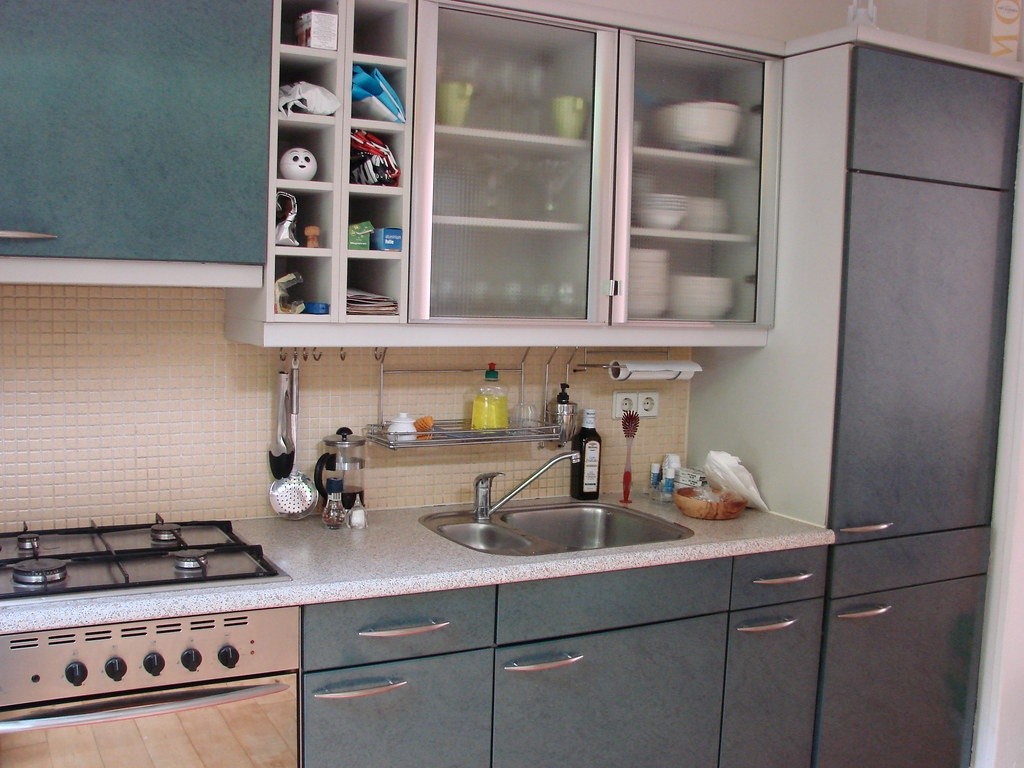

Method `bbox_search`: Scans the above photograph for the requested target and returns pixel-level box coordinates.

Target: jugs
[313,426,366,515]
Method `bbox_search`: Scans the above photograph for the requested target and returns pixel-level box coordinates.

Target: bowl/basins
[632,192,731,232]
[628,248,734,319]
[648,100,743,152]
[674,487,748,519]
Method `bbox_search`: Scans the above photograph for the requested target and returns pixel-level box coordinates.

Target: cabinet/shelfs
[0,0,1024,768]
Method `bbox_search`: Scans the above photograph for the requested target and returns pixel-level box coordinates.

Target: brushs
[622,410,640,502]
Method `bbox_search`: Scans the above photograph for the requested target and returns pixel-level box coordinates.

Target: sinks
[499,505,690,548]
[419,510,536,557]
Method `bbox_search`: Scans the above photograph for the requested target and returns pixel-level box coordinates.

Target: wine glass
[434,147,578,219]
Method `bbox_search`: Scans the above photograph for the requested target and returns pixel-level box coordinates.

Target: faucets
[470,448,582,520]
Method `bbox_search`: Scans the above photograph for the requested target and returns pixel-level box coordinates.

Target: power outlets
[637,389,659,419]
[612,391,638,419]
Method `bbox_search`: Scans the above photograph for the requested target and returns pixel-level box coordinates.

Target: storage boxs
[293,9,339,52]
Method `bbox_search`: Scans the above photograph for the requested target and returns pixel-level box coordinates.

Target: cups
[551,96,586,140]
[436,81,474,128]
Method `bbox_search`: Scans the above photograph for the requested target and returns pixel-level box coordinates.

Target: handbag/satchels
[278,82,341,117]
[351,64,405,125]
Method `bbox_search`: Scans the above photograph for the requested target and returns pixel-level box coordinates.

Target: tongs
[268,370,296,479]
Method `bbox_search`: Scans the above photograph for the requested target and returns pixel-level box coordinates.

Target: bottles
[347,494,369,529]
[387,411,418,443]
[321,476,346,530]
[650,462,661,487]
[569,408,602,500]
[664,469,675,493]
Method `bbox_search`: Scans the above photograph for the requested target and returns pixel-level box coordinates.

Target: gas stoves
[0,513,293,607]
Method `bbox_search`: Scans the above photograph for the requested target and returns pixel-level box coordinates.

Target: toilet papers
[607,359,704,381]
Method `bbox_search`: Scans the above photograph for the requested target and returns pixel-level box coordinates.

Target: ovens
[0,603,304,768]
